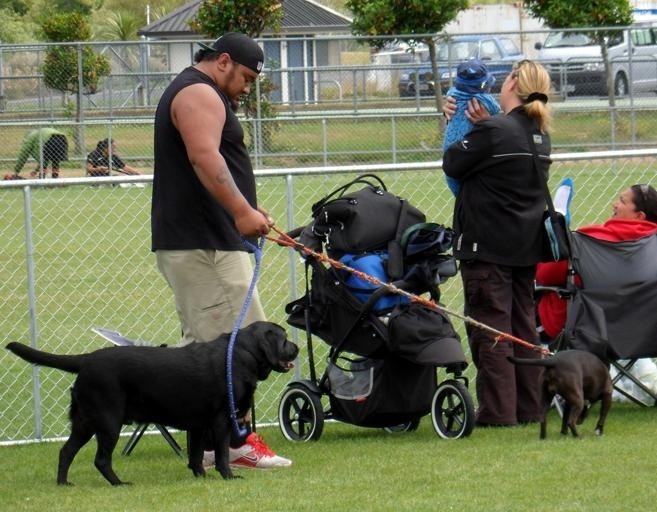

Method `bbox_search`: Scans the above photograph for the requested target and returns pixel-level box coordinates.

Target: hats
[452,58,497,93]
[195,30,264,75]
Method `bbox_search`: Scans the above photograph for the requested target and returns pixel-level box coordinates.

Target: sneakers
[201,432,292,471]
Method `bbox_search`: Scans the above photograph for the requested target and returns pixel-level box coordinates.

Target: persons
[535,181,657,340]
[12,126,70,178]
[86,138,142,186]
[151,31,294,469]
[445,58,503,197]
[440,60,556,428]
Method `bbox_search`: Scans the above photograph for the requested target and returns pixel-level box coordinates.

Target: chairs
[90,325,184,461]
[535,229,657,409]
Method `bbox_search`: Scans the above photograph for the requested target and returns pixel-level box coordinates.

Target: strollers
[278,225,477,446]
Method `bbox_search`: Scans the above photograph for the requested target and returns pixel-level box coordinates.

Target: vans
[533,20,657,96]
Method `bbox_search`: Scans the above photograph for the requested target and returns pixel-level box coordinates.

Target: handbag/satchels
[538,202,571,262]
[302,172,449,317]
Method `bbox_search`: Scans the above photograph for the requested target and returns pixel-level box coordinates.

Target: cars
[399,37,524,97]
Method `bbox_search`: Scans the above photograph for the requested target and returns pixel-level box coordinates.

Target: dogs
[4,320,299,487]
[505,349,614,440]
